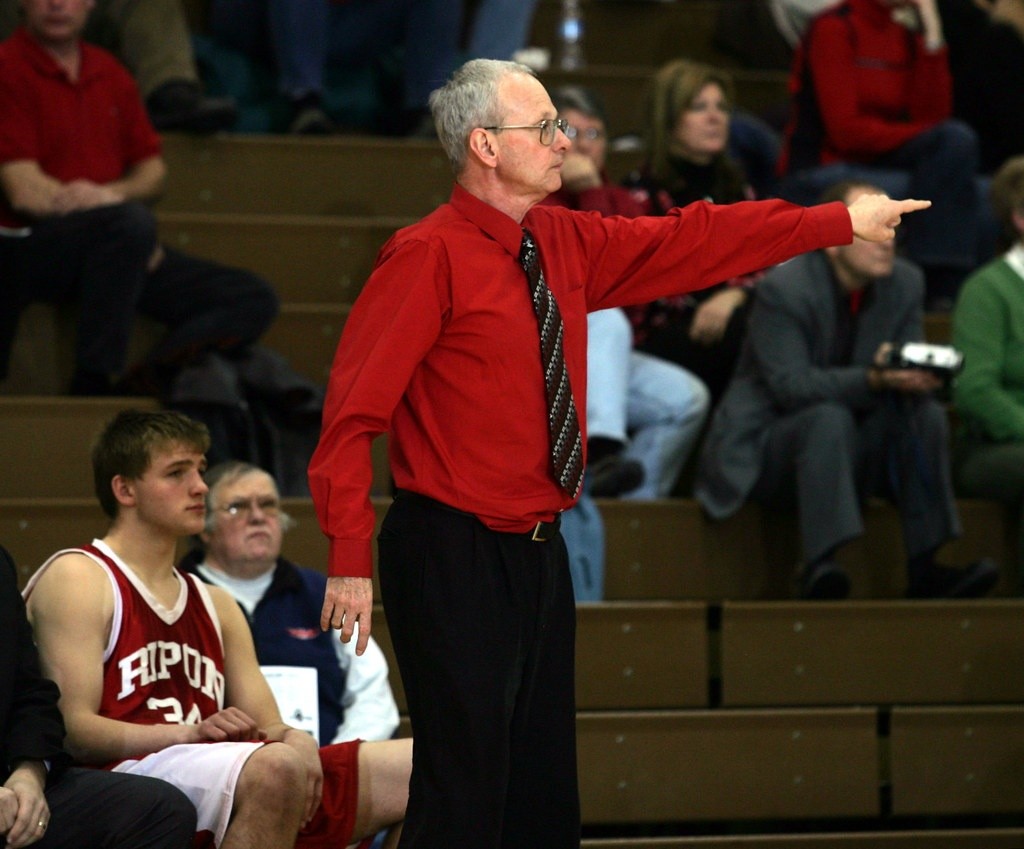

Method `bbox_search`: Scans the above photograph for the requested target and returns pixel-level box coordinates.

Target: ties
[520,225,584,500]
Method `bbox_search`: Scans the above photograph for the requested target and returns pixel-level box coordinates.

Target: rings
[38,821,46,829]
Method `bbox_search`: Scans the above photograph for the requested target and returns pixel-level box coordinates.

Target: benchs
[0,0,1024,849]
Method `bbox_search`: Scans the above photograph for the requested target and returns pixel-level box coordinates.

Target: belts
[530,518,562,542]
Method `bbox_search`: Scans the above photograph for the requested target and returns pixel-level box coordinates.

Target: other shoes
[903,558,1004,597]
[590,455,646,500]
[809,563,876,599]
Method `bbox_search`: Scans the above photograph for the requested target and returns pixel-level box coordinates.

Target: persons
[172,455,400,750]
[772,0,1024,320]
[535,79,711,501]
[0,0,278,390]
[107,1,227,134]
[307,57,935,849]
[249,0,537,134]
[941,158,1024,534]
[18,403,415,849]
[630,54,777,400]
[0,543,198,849]
[682,170,997,602]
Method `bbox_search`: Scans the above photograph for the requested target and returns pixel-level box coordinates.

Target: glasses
[479,118,570,146]
[209,500,281,521]
